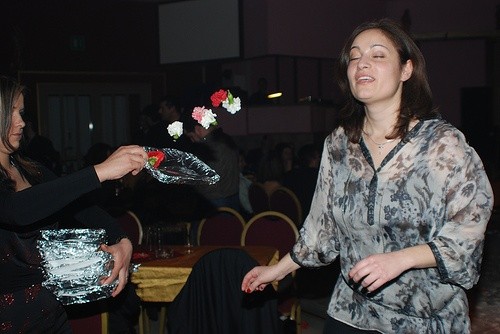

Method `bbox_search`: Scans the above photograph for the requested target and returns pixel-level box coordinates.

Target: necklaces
[366,133,399,155]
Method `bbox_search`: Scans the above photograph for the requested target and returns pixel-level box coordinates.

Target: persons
[27,92,318,198]
[242,20,495,334]
[0,72,148,334]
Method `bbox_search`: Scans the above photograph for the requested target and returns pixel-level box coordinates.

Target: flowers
[192,106,218,129]
[210,88,242,115]
[147,151,165,169]
[167,121,184,139]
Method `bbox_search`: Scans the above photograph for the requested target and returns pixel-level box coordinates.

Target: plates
[37,227,113,305]
[142,145,221,185]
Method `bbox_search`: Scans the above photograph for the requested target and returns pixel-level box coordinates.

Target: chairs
[67,298,108,334]
[118,183,302,334]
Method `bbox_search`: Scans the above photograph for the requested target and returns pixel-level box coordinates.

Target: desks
[129,243,280,334]
